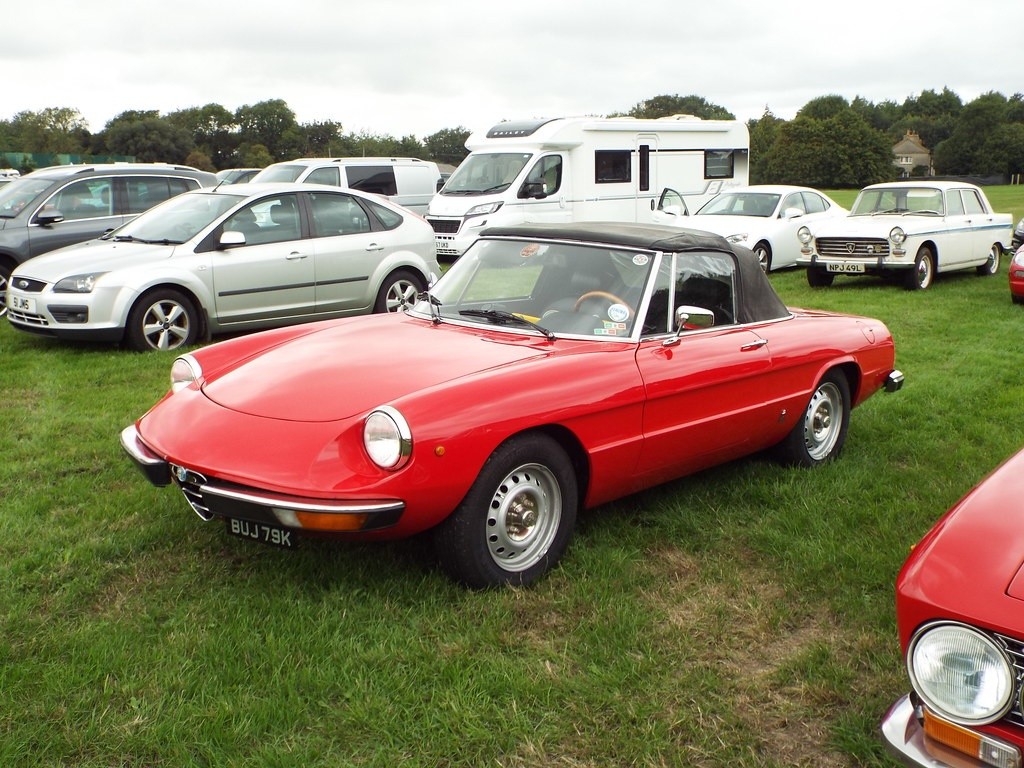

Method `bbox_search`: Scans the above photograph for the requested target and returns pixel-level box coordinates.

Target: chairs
[642,277,733,332]
[225,204,300,233]
[743,197,798,220]
[500,160,528,193]
[58,182,95,219]
[540,270,624,323]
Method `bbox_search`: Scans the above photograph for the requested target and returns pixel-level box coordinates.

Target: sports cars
[121,222,903,595]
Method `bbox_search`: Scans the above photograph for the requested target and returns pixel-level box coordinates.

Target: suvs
[0,162,221,308]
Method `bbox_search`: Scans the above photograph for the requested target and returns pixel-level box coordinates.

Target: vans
[213,167,292,187]
[229,157,445,228]
[418,113,750,255]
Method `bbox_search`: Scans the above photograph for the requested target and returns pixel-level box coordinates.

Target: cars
[75,184,148,212]
[0,168,20,178]
[791,181,1016,292]
[654,185,851,277]
[5,182,441,355]
[879,446,1024,768]
